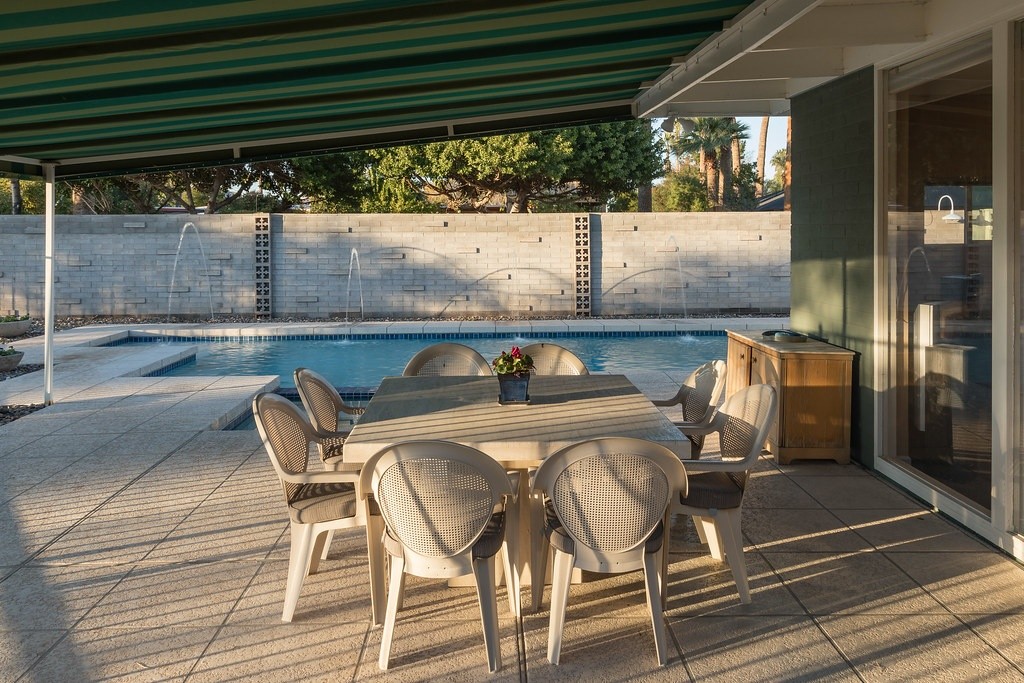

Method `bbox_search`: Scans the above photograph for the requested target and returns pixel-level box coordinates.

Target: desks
[343,375,691,586]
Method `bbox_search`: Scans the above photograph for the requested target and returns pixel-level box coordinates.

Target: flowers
[492,346,536,376]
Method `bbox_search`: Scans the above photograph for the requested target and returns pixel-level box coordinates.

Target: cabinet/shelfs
[725,329,856,465]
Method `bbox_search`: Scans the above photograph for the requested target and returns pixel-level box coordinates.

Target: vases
[496,370,531,407]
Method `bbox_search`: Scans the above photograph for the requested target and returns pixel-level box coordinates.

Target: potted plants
[0,315,32,338]
[0,346,24,371]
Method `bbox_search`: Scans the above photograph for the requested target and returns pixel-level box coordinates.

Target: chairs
[528,436,689,667]
[521,344,590,374]
[253,392,391,628]
[652,360,727,461]
[402,343,494,376]
[295,367,366,560]
[359,438,521,674]
[657,383,776,605]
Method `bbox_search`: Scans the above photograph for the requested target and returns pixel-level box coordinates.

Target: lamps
[938,195,962,224]
